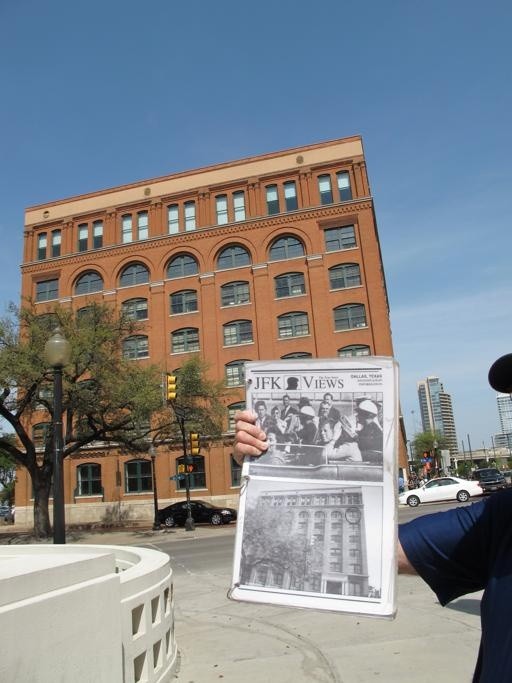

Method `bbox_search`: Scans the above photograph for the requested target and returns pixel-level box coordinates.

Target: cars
[471,468,512,492]
[398,477,483,507]
[160,500,237,526]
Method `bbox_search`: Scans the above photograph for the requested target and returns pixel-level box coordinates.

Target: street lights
[432,439,441,477]
[44,326,71,545]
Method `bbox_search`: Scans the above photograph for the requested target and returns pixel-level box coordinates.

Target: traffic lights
[164,373,179,402]
[190,433,202,457]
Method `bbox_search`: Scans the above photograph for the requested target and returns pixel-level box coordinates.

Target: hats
[489,353,512,393]
[354,400,378,415]
[299,406,316,416]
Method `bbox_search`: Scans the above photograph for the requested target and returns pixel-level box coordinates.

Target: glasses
[272,411,279,415]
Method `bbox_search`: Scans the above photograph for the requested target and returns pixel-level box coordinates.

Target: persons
[229,353,512,683]
[399,468,472,494]
[248,391,382,468]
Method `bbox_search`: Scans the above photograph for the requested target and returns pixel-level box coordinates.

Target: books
[228,357,401,622]
